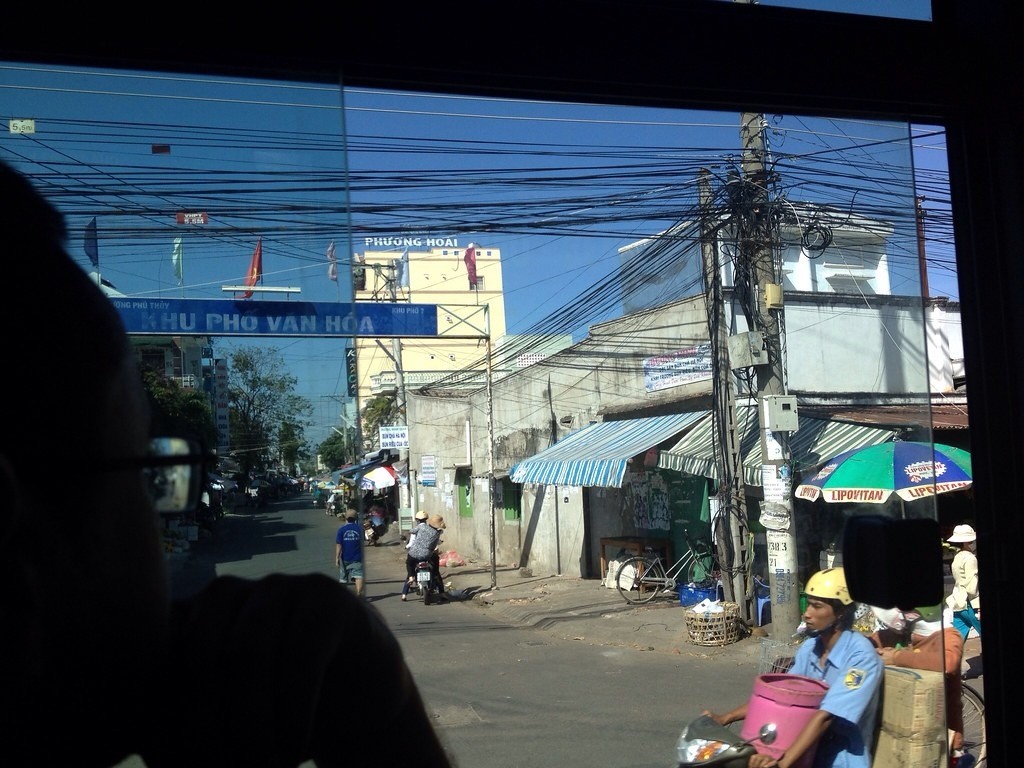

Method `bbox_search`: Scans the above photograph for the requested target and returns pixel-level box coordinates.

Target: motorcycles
[401,536,440,605]
[361,515,380,546]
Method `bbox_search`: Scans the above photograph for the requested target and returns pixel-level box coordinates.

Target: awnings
[658,406,895,488]
[509,410,712,488]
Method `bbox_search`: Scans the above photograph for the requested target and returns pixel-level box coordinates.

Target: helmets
[803,565,855,605]
[415,511,428,520]
[914,601,942,623]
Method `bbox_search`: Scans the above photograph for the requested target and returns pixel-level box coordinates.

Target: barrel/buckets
[741,673,829,767]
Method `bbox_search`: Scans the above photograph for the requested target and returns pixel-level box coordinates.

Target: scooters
[672,714,777,768]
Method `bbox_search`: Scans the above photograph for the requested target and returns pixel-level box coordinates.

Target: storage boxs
[871,727,949,768]
[677,582,724,608]
[881,664,947,742]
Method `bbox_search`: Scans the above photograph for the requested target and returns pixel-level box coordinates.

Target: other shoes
[407,580,413,585]
[401,596,406,601]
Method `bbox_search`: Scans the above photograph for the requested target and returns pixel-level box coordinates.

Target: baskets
[683,600,741,646]
[757,636,799,675]
[692,534,712,559]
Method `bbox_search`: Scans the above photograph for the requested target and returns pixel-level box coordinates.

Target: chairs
[755,576,771,627]
[398,508,412,535]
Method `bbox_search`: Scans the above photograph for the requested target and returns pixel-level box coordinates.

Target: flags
[236,238,262,298]
[172,237,183,278]
[397,250,409,287]
[84,217,98,266]
[464,248,476,284]
[327,242,337,281]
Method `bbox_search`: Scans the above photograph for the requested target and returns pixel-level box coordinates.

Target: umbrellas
[361,465,398,490]
[795,437,973,519]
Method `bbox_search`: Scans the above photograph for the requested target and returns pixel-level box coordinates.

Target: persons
[0,160,450,768]
[401,511,446,600]
[875,617,964,768]
[700,567,885,768]
[947,524,981,642]
[335,508,365,596]
[326,493,338,515]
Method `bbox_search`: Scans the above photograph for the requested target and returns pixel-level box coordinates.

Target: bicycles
[615,528,719,604]
[756,638,987,768]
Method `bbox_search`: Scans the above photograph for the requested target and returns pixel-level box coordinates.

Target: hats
[429,515,446,531]
[947,524,977,543]
[346,507,357,520]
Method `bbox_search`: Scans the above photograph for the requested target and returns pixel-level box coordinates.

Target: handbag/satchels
[945,585,968,612]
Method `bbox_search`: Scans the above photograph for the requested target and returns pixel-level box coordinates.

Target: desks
[599,537,673,595]
